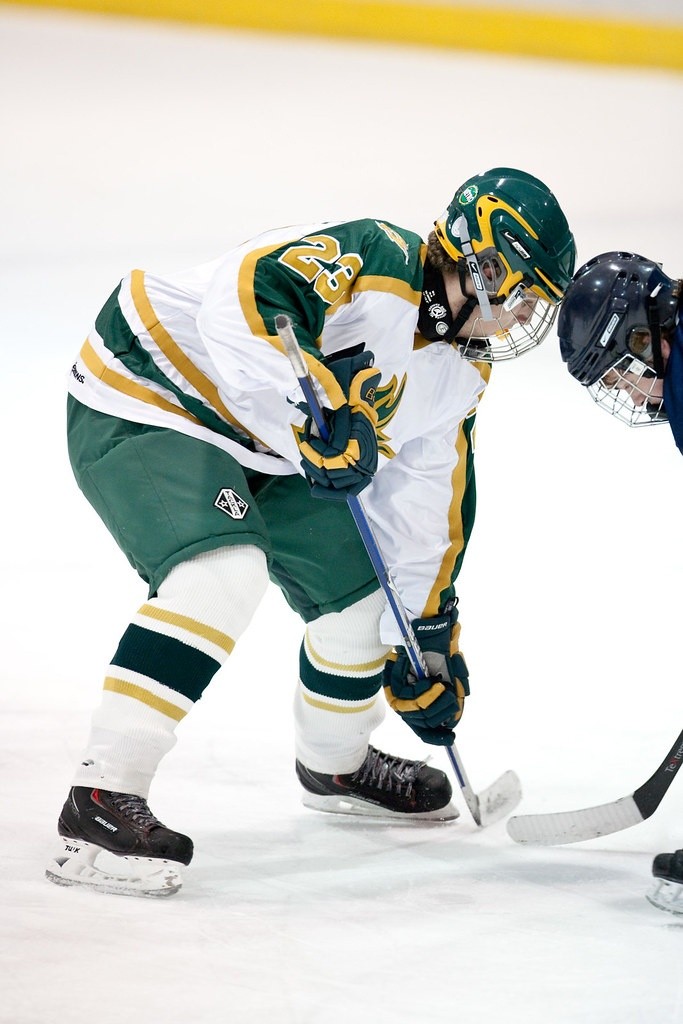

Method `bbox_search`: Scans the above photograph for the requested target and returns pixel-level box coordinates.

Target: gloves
[290,351,380,501]
[382,596,469,748]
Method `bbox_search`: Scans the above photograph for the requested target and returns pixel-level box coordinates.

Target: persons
[557,251,683,884]
[58,167,575,863]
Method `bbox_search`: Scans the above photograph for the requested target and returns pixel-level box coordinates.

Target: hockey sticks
[271,308,524,834]
[503,727,683,851]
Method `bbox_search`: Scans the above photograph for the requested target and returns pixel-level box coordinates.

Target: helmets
[436,168,576,362]
[557,251,680,428]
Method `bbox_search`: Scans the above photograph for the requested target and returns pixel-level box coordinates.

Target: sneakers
[45,787,193,900]
[296,745,460,821]
[646,850,683,918]
